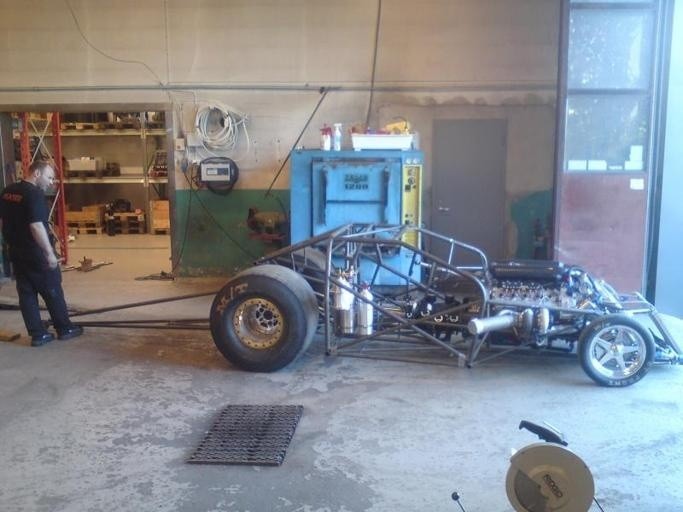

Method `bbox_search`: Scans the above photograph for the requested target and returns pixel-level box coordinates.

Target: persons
[0,160,83,347]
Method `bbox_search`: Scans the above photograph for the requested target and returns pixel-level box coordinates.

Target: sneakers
[31,330,54,346]
[58,325,83,340]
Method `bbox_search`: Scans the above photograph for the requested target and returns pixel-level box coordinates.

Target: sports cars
[208,219,683,388]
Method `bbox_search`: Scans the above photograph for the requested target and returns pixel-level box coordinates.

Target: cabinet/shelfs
[11,112,170,235]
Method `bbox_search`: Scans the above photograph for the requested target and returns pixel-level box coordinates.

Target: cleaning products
[334,123,341,151]
[320,124,332,151]
